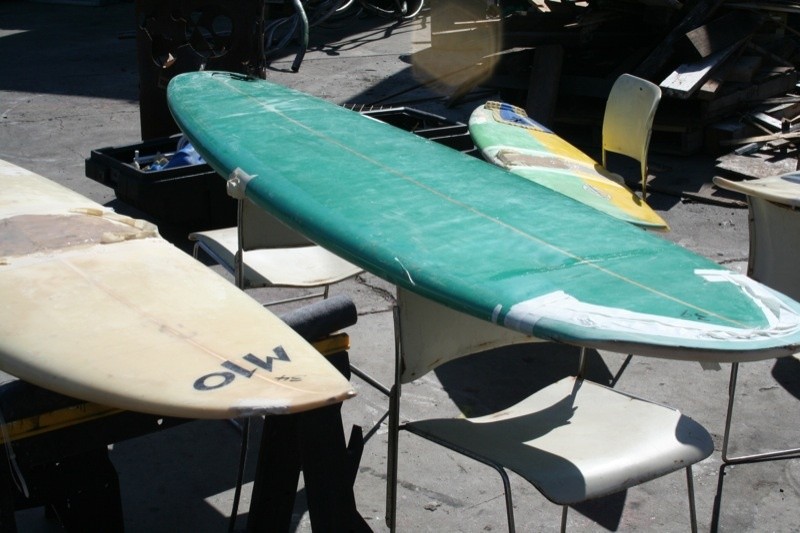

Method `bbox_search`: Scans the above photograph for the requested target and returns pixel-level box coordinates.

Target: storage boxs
[85,106,482,249]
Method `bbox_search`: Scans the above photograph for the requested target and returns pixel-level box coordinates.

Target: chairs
[384,283,714,533]
[603,73,662,201]
[189,174,366,304]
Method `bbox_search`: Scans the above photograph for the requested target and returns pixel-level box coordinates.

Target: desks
[713,169,800,303]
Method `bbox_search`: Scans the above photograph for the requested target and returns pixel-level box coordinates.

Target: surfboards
[167,71,800,363]
[1,156,359,419]
[468,102,668,232]
